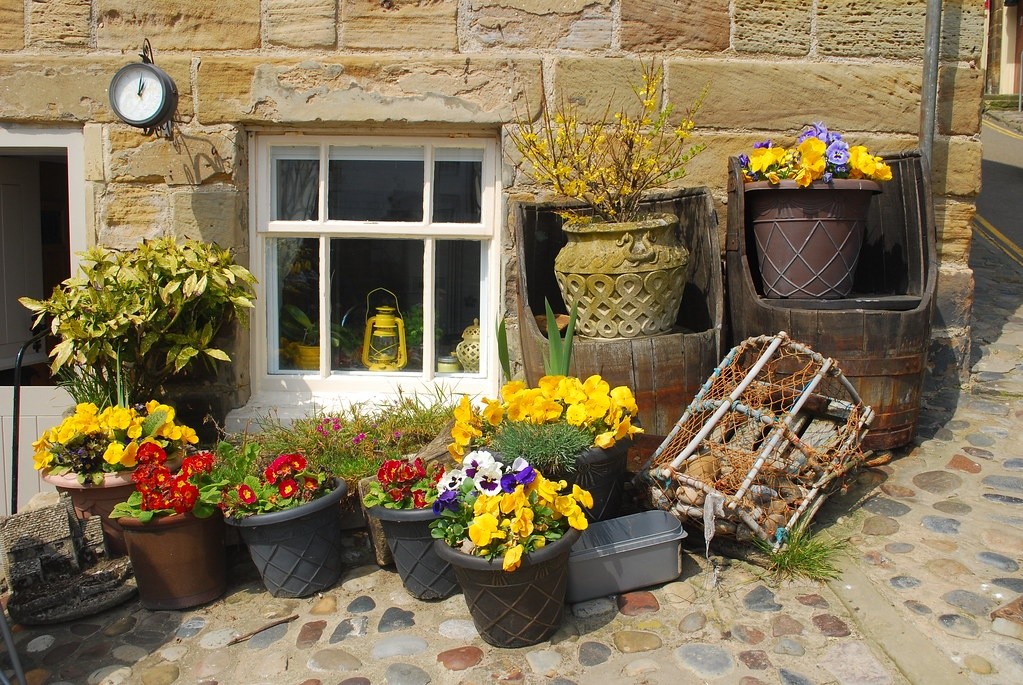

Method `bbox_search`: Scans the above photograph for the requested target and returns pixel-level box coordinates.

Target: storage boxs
[561,510,689,603]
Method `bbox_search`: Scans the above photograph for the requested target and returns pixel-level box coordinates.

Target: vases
[555,422,640,520]
[371,506,461,601]
[555,211,693,337]
[43,446,186,552]
[745,178,881,300]
[224,477,344,599]
[115,508,224,610]
[434,518,582,647]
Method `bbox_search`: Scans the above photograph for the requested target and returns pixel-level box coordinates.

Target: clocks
[107,63,179,131]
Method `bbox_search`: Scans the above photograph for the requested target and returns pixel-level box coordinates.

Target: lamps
[361,287,408,372]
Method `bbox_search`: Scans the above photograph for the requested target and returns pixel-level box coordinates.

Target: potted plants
[297,322,321,370]
[16,233,258,446]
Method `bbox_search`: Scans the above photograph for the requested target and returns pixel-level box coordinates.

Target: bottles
[437,356,459,373]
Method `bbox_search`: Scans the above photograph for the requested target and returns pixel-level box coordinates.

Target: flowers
[508,47,715,226]
[221,453,338,520]
[733,124,896,185]
[31,401,200,485]
[445,373,648,475]
[428,445,592,570]
[107,438,220,526]
[362,457,450,511]
